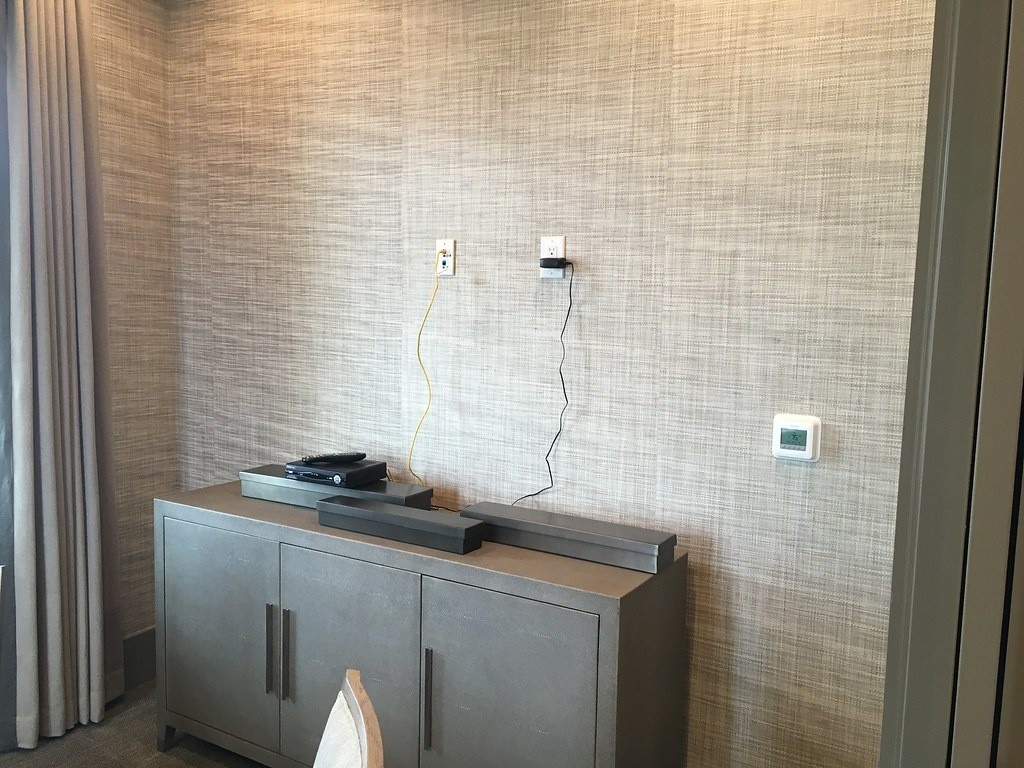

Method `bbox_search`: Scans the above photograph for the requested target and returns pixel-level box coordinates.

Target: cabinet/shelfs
[154,479,688,768]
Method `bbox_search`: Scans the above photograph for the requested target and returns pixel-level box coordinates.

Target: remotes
[302,452,367,464]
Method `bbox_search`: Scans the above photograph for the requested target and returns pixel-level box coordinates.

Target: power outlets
[540,235,566,279]
[435,238,455,276]
